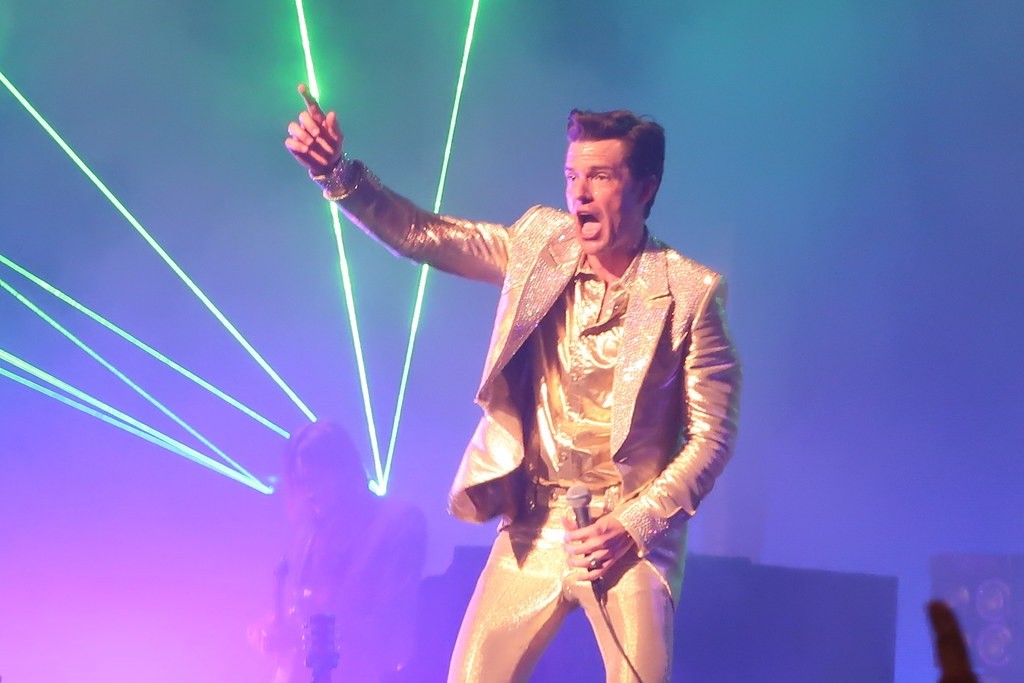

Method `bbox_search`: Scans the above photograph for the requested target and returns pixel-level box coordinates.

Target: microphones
[566,484,607,602]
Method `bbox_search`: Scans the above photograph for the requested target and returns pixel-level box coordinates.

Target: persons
[246,419,426,682]
[282,82,739,682]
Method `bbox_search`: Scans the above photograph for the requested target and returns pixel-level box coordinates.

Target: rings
[587,554,600,569]
